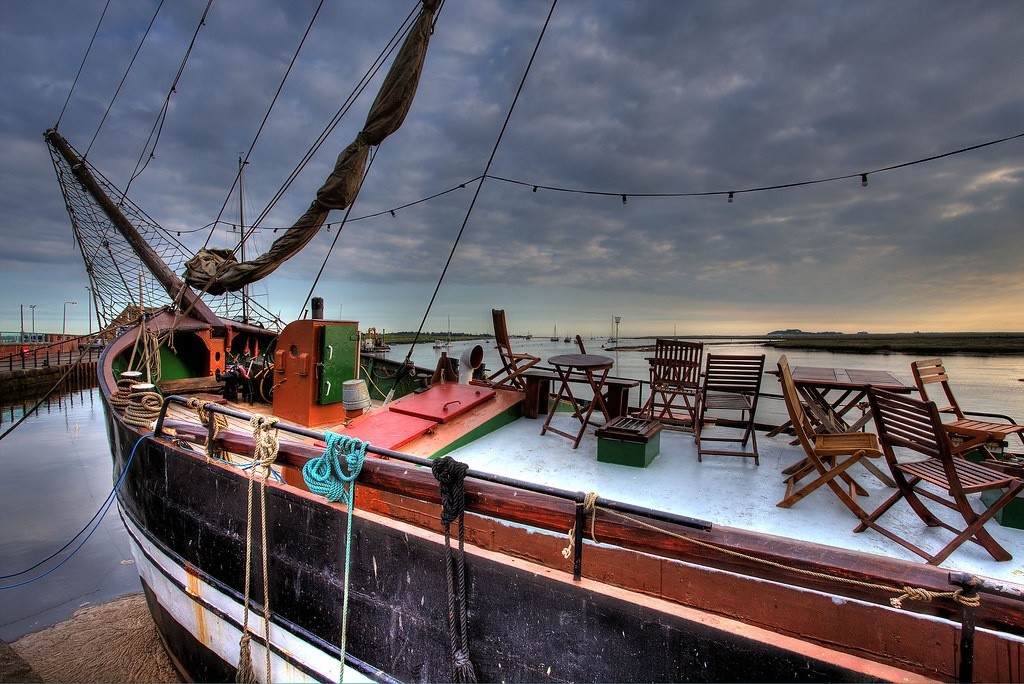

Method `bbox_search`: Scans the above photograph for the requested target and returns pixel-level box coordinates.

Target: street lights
[85,286,92,334]
[30,304,37,333]
[62,302,78,354]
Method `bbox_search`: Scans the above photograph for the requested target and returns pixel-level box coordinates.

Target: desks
[778,366,922,488]
[539,354,615,449]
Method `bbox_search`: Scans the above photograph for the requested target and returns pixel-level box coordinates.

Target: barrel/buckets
[341,379,373,420]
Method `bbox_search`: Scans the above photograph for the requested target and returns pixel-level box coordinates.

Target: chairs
[636,337,766,467]
[776,354,885,520]
[911,358,1024,498]
[853,384,1024,566]
[488,308,542,389]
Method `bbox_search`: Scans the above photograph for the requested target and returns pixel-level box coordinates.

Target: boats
[551,324,559,342]
[361,327,391,353]
[564,330,572,343]
[432,339,453,350]
[574,339,585,344]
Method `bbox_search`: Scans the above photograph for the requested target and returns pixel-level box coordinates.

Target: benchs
[522,369,640,422]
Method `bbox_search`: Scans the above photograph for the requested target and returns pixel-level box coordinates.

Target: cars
[78,333,109,352]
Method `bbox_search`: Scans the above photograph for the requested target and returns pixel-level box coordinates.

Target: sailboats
[42,2,1024,684]
[606,314,617,343]
